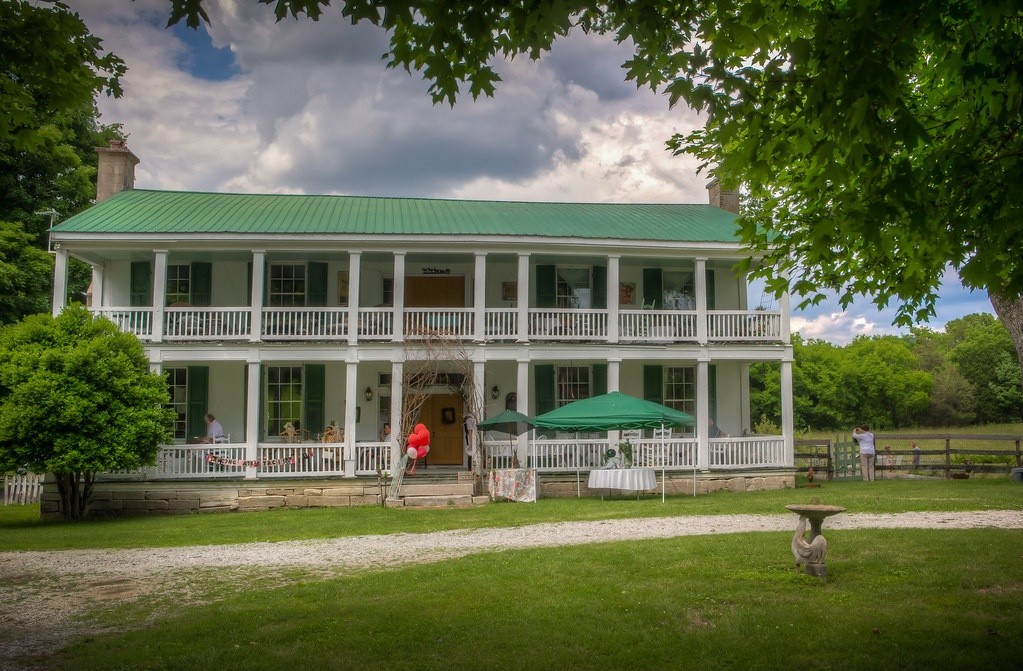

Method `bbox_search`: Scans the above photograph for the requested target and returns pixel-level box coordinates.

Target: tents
[534,392,697,504]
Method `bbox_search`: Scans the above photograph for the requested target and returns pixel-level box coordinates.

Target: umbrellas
[476,409,535,468]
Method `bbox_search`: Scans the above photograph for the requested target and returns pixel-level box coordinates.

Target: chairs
[483,435,495,441]
[212,434,231,444]
[279,427,342,443]
[622,429,641,439]
[653,428,672,439]
[537,434,547,440]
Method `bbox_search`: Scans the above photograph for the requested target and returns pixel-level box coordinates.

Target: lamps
[365,388,372,401]
[492,385,499,398]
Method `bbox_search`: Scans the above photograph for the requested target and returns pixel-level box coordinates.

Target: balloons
[407,423,430,460]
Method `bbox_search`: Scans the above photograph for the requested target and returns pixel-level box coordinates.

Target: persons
[202,412,225,444]
[911,440,920,471]
[358,424,391,470]
[852,425,875,483]
[883,445,895,473]
[676,418,727,457]
[320,426,333,442]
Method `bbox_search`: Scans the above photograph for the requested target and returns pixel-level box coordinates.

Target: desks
[588,468,657,501]
[487,468,539,503]
